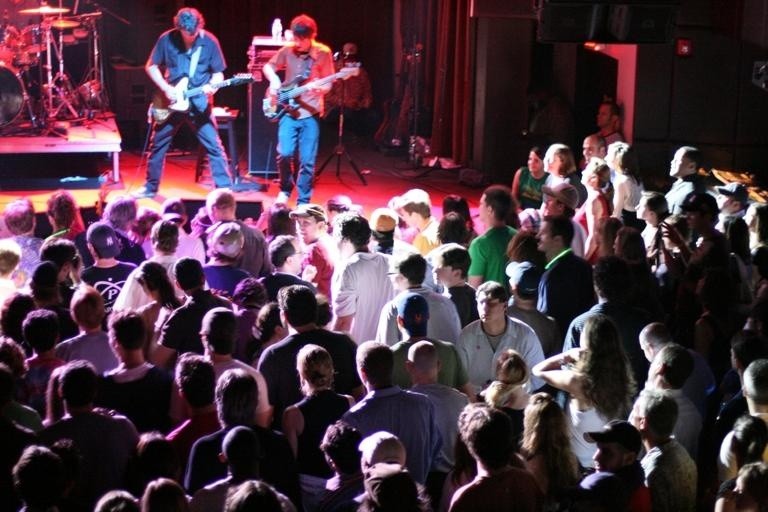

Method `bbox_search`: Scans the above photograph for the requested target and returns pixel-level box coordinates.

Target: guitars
[372,44,424,148]
[261,61,362,123]
[151,73,253,124]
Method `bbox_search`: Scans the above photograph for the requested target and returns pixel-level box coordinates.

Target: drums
[0,61,26,128]
[79,80,104,101]
[63,34,80,45]
[73,28,88,40]
[20,25,48,54]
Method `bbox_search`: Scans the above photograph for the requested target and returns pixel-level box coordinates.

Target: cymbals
[49,20,80,29]
[18,5,70,15]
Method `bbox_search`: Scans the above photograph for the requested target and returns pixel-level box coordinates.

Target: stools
[195,108,240,182]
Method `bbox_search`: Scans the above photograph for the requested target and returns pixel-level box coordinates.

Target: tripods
[314,93,369,187]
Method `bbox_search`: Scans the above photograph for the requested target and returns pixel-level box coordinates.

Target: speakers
[180,199,263,223]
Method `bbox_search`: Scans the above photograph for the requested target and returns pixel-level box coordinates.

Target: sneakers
[275,192,288,206]
[134,190,154,200]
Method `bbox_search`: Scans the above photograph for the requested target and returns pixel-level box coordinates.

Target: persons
[262,12,335,205]
[574,100,624,172]
[1,135,766,511]
[132,7,241,199]
[323,38,373,127]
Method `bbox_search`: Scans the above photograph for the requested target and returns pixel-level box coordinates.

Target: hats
[33,261,56,282]
[200,278,267,338]
[369,208,398,232]
[211,222,246,258]
[583,418,640,454]
[715,182,750,200]
[87,222,120,259]
[504,261,541,289]
[288,203,327,223]
[357,429,405,467]
[161,199,186,223]
[397,291,430,322]
[541,182,579,208]
[196,207,211,226]
